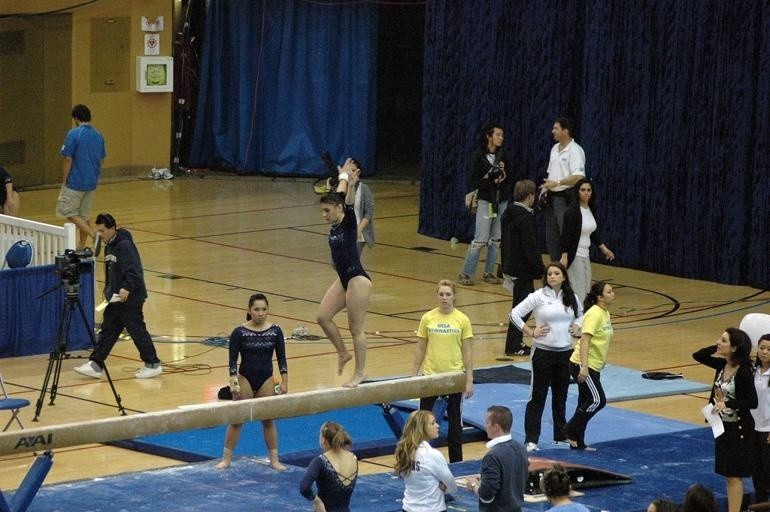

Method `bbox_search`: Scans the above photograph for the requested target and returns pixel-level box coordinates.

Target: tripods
[32,284,131,417]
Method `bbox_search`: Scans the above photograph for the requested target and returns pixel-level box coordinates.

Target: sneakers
[159,168,174,181]
[481,271,503,284]
[456,273,474,285]
[74,360,104,379]
[135,365,161,379]
[526,441,539,452]
[506,349,531,356]
[521,341,530,348]
[552,436,571,446]
[92,231,103,257]
[147,166,161,180]
[75,247,85,255]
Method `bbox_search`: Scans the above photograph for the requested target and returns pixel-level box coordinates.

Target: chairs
[0,240,34,269]
[0,374,30,430]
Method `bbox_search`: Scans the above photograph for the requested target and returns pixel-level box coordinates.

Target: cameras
[55,247,93,279]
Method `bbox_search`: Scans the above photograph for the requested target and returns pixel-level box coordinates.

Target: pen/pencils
[713,396,723,417]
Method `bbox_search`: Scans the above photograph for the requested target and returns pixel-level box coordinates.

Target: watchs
[472,483,477,492]
[557,180,560,188]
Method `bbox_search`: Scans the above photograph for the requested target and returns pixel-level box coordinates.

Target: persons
[73,212,164,378]
[299,421,358,511]
[510,264,582,452]
[56,105,106,260]
[749,333,766,509]
[338,162,374,273]
[693,328,759,512]
[562,283,615,452]
[461,124,505,286]
[216,294,288,472]
[684,484,716,511]
[649,499,675,511]
[468,406,528,512]
[537,118,585,262]
[502,180,545,355]
[393,410,458,511]
[539,468,606,512]
[317,158,372,389]
[0,169,19,218]
[412,279,472,463]
[559,180,615,312]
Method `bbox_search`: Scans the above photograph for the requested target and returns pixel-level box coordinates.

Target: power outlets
[206,336,226,346]
